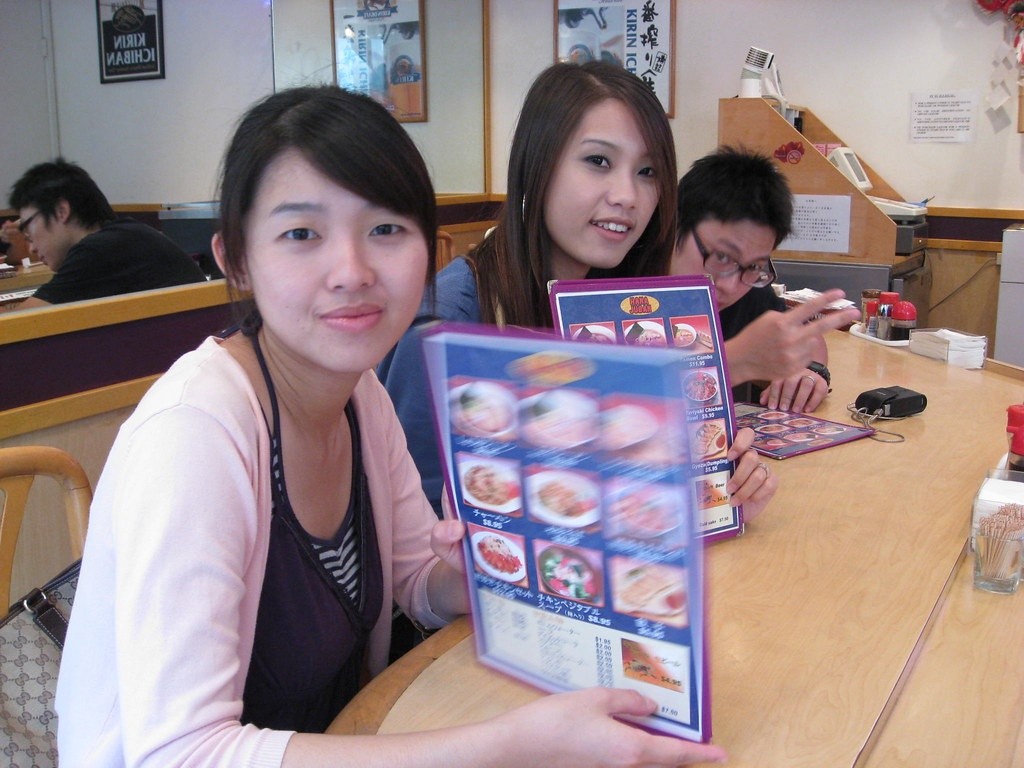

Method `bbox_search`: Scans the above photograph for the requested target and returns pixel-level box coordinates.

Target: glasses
[688,222,778,288]
[18,201,53,238]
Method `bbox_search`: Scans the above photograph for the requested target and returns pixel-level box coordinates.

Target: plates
[614,558,687,614]
[525,469,601,527]
[625,321,668,348]
[538,545,601,601]
[571,325,616,344]
[470,530,526,582]
[449,382,518,439]
[605,482,686,538]
[601,403,659,450]
[672,323,697,347]
[519,388,600,449]
[692,422,726,461]
[457,461,521,514]
[682,370,718,402]
[753,411,847,446]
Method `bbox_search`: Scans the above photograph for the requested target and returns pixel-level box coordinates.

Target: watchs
[807,361,832,393]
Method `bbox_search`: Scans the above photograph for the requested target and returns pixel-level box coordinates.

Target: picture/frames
[330,0,427,123]
[95,0,165,83]
[553,0,676,118]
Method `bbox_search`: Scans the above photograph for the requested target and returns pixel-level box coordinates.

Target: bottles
[1006,405,1024,449]
[865,301,879,332]
[861,288,883,333]
[890,300,917,341]
[877,304,892,340]
[879,292,900,307]
[1008,429,1024,483]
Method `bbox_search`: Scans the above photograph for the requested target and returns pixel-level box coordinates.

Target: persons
[0,159,225,400]
[667,148,860,414]
[56,90,724,768]
[376,59,779,525]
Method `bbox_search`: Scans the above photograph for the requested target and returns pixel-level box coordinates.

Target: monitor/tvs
[825,147,873,193]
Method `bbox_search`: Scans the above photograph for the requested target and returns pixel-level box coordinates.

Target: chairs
[0,445,91,618]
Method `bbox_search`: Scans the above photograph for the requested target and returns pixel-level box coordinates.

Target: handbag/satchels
[0,556,82,768]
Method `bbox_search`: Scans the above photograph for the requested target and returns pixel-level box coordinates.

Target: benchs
[0,279,256,606]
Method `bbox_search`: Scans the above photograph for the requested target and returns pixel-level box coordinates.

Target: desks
[325,328,1023,768]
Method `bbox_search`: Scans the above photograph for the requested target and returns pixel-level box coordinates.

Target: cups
[974,536,1023,595]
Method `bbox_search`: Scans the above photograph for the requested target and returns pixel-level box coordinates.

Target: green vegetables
[542,554,592,598]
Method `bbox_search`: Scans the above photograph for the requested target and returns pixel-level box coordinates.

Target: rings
[808,376,815,383]
[758,463,770,477]
